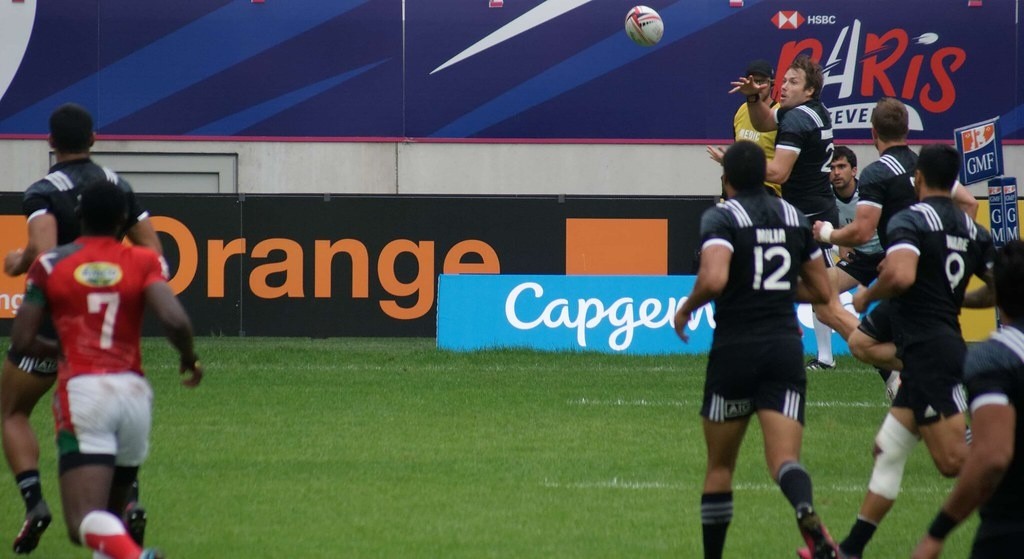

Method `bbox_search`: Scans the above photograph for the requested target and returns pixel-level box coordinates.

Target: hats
[746,59,771,78]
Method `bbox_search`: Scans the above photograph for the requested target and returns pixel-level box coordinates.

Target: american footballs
[625,5,664,47]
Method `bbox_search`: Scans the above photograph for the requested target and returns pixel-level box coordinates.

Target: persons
[675,54,1024,559]
[0,104,204,559]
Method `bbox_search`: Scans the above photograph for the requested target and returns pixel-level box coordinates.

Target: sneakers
[127,504,147,547]
[886,372,902,401]
[805,359,837,372]
[797,505,841,559]
[796,545,861,559]
[139,547,164,559]
[13,501,52,554]
